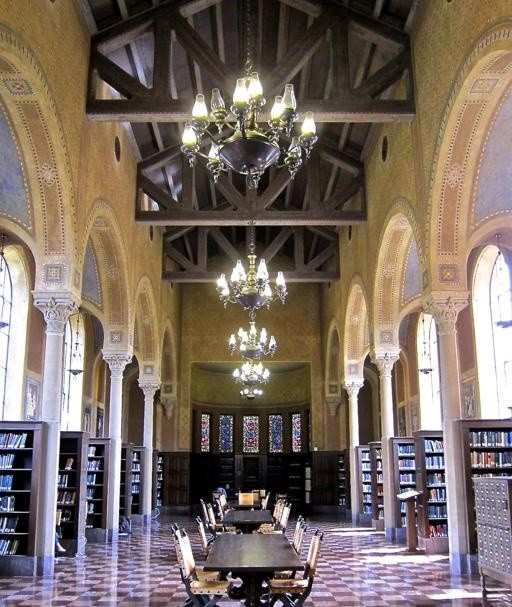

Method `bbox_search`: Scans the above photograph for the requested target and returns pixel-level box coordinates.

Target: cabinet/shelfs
[3,421,165,577]
[356,417,511,594]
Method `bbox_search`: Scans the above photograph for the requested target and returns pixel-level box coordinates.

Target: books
[361,452,372,513]
[376,449,385,519]
[397,445,417,526]
[57,458,76,539]
[131,452,140,493]
[424,440,448,537]
[86,446,101,528]
[156,456,163,506]
[339,457,346,506]
[471,431,512,477]
[0,433,28,554]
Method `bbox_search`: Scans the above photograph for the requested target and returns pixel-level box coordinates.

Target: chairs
[167,485,324,606]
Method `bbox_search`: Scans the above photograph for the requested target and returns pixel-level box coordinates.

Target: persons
[56,536,67,554]
